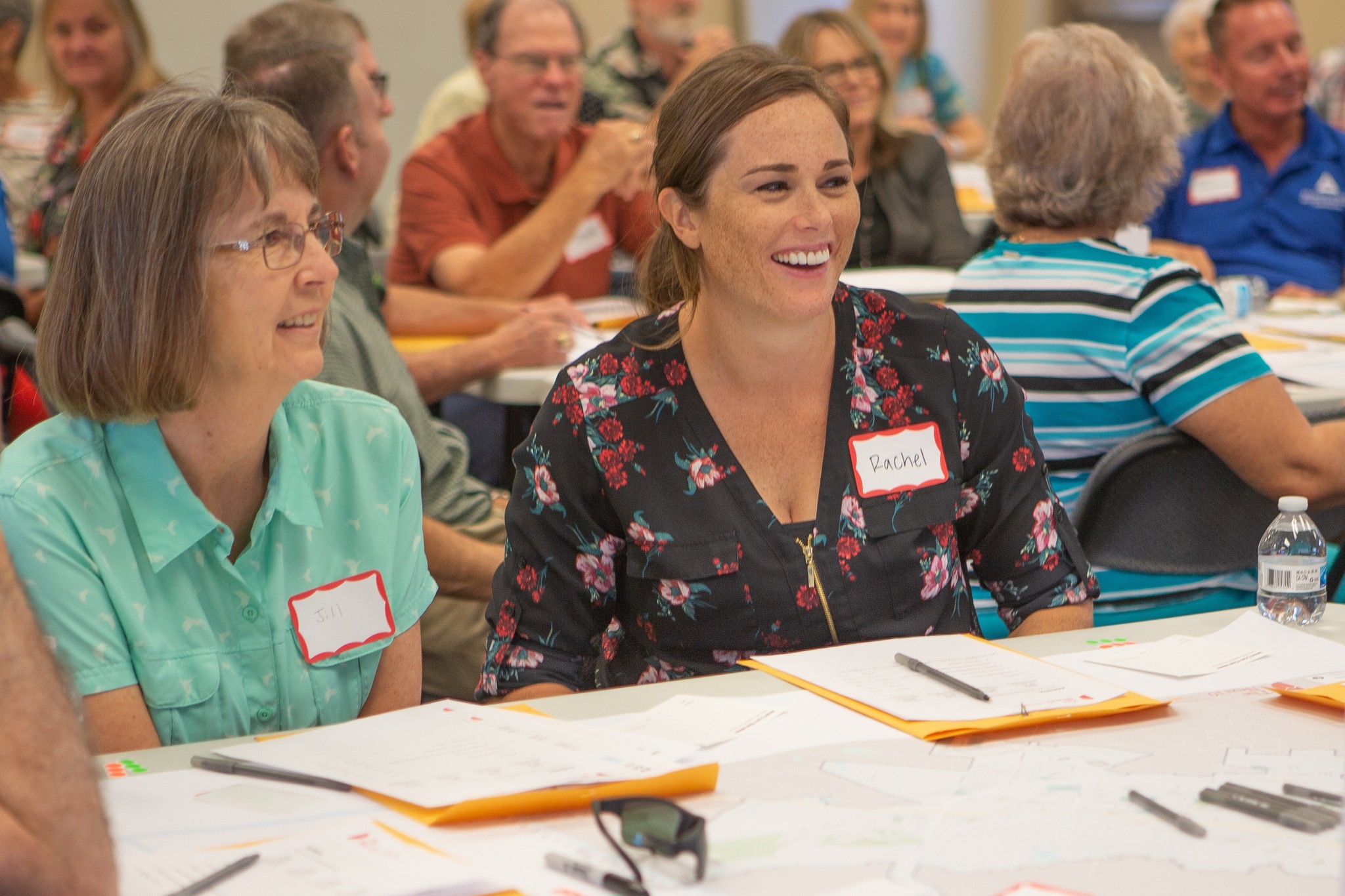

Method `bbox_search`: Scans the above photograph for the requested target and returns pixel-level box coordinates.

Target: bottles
[1256,495,1327,623]
[1216,274,1264,311]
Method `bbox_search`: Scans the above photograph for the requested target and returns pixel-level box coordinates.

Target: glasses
[367,72,388,96]
[591,793,708,890]
[210,209,344,271]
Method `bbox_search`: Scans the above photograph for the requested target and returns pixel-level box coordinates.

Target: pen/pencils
[520,307,602,338]
[1130,788,1207,840]
[1202,781,1340,833]
[192,753,353,792]
[171,851,261,894]
[545,853,646,894]
[893,650,989,700]
[1282,784,1344,808]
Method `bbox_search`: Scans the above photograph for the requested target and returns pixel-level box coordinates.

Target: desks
[102,602,1345,896]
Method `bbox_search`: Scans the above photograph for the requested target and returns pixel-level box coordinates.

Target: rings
[557,332,573,352]
[630,129,643,143]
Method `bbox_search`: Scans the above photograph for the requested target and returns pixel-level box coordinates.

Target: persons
[0,522,117,896]
[1,84,440,755]
[469,48,1102,708]
[1139,0,1345,299]
[943,20,1345,640]
[776,0,987,272]
[0,0,737,709]
